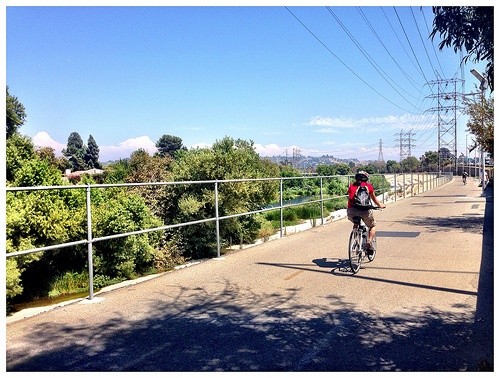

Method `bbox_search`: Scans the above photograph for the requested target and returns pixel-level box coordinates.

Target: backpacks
[350,182,373,210]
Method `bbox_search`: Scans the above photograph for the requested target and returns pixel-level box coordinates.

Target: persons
[479,169,489,187]
[346,171,387,252]
[461,170,468,183]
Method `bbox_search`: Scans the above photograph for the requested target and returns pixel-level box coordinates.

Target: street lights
[469,68,490,195]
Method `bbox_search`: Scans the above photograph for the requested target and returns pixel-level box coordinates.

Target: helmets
[355,170,369,182]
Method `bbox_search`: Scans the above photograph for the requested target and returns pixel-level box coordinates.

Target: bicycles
[462,174,468,185]
[348,206,385,274]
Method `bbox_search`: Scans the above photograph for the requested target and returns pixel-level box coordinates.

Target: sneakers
[367,240,373,250]
